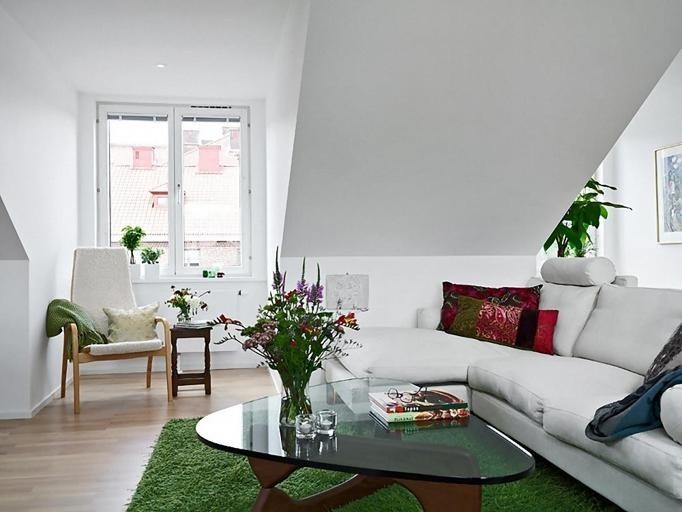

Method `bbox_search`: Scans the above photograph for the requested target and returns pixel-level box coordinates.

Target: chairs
[59,248,174,416]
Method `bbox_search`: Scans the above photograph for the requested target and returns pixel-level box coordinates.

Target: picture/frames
[654,141,682,246]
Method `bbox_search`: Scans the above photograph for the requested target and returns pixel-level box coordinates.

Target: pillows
[446,296,484,337]
[102,301,162,342]
[436,281,543,335]
[473,301,559,356]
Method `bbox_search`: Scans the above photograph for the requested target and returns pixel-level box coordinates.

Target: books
[369,410,469,433]
[367,389,470,423]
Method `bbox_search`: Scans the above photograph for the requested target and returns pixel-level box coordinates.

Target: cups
[316,410,339,436]
[296,441,338,460]
[296,413,318,440]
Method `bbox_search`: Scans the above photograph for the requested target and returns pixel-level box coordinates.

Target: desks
[170,324,212,398]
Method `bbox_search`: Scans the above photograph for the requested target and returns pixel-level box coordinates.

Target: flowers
[164,284,211,316]
[206,244,362,423]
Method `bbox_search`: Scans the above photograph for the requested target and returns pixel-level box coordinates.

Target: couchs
[320,285,681,511]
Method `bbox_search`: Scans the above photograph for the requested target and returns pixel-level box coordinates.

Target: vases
[176,308,191,325]
[278,371,313,427]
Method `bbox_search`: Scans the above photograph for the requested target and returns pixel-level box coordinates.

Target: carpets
[124,410,624,510]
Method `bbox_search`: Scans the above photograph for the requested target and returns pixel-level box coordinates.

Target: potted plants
[121,224,146,279]
[140,248,166,280]
